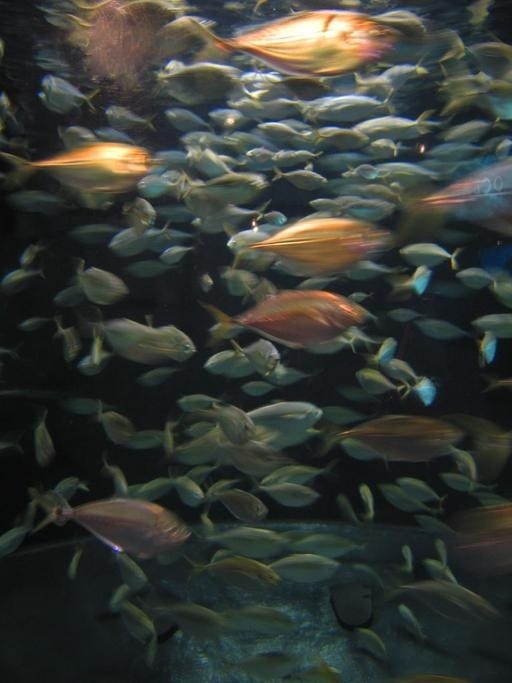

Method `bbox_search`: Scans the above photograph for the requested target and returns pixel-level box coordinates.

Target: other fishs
[0,0,512,683]
